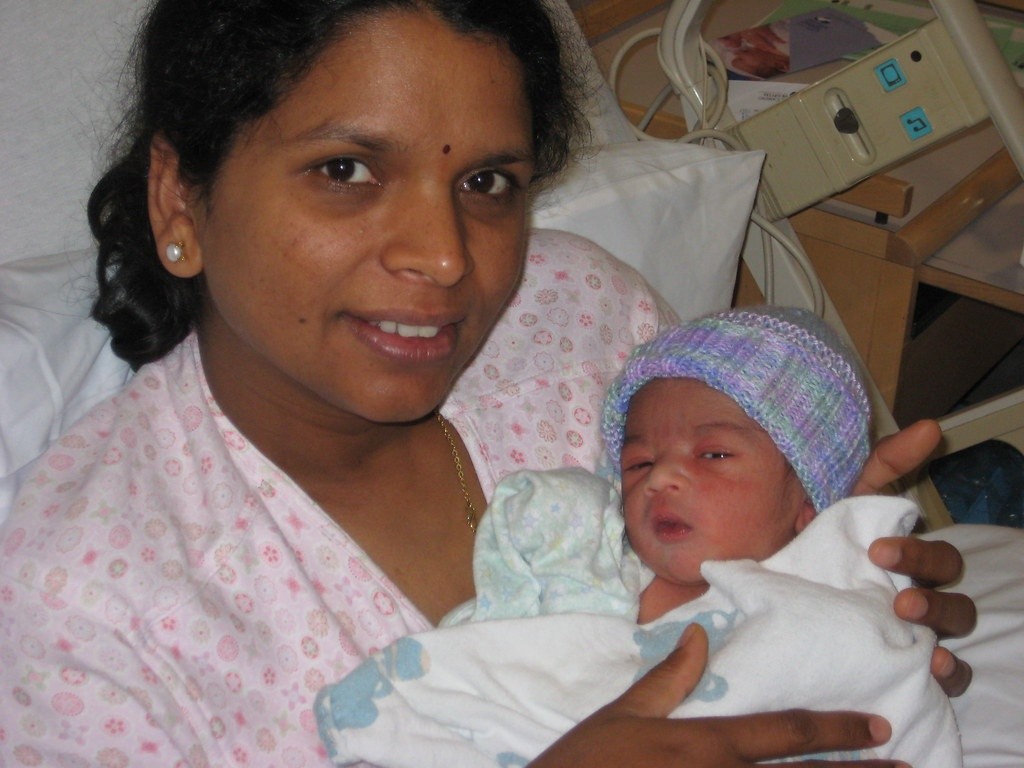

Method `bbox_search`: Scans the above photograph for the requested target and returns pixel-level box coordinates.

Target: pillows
[3,141,766,481]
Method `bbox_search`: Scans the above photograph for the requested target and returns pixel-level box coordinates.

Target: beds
[0,1,1024,767]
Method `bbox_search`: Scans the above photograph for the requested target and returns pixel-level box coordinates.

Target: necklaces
[435,409,479,533]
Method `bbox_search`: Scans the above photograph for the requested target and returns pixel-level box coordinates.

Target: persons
[312,303,959,768]
[715,24,788,80]
[0,0,979,768]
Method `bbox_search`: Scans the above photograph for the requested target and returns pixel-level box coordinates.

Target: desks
[587,1,1024,530]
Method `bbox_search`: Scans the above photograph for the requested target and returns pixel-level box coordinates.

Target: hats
[600,304,872,516]
